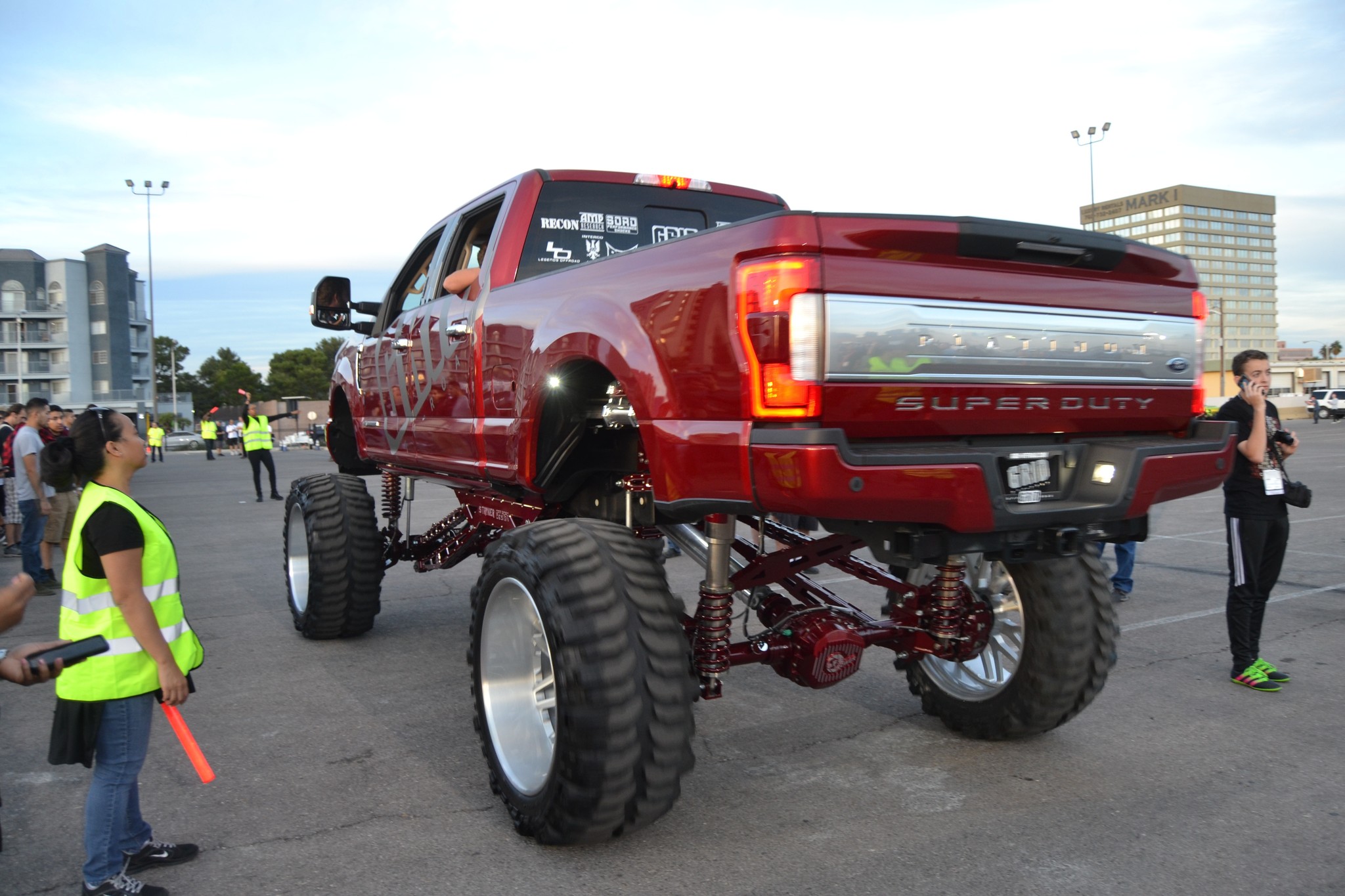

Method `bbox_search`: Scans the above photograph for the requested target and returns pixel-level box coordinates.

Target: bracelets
[0,648,9,662]
[246,399,250,402]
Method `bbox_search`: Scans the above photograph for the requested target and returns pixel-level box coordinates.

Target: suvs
[1307,388,1345,419]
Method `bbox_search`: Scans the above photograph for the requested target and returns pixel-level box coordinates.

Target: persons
[0,401,98,597]
[362,379,519,468]
[777,513,820,577]
[200,411,218,460]
[242,391,303,502]
[442,241,491,293]
[1090,540,1137,603]
[1307,396,1320,424]
[0,567,89,690]
[225,419,239,455]
[236,416,248,458]
[1327,393,1341,424]
[41,400,208,896]
[661,535,683,559]
[1210,347,1302,694]
[215,420,225,457]
[751,512,784,553]
[146,421,166,462]
[318,280,345,309]
[326,311,345,325]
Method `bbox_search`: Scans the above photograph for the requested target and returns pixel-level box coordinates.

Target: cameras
[1273,429,1294,445]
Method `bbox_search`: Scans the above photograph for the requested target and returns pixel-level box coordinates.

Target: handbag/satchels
[1281,478,1313,508]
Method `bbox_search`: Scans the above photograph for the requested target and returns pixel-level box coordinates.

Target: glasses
[248,407,259,410]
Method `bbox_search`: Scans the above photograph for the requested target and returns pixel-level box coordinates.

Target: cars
[165,431,206,450]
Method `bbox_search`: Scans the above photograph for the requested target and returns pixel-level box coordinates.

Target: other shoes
[1313,422,1318,424]
[229,451,241,455]
[152,458,163,463]
[1331,420,1341,424]
[241,455,247,459]
[217,453,224,456]
[209,457,215,460]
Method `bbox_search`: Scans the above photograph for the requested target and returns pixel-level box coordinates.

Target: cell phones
[1237,376,1265,395]
[25,633,109,674]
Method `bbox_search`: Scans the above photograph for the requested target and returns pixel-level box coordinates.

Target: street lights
[1071,123,1111,232]
[169,337,183,430]
[125,179,170,425]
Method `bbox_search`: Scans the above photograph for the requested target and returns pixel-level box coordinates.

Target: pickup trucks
[280,167,1240,848]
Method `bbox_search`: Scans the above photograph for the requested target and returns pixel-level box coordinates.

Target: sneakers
[30,584,54,596]
[42,579,63,589]
[0,532,7,545]
[122,838,200,877]
[81,875,171,896]
[4,541,24,557]
[1109,588,1129,602]
[256,497,262,502]
[1253,658,1291,682]
[270,493,283,500]
[1230,664,1283,692]
